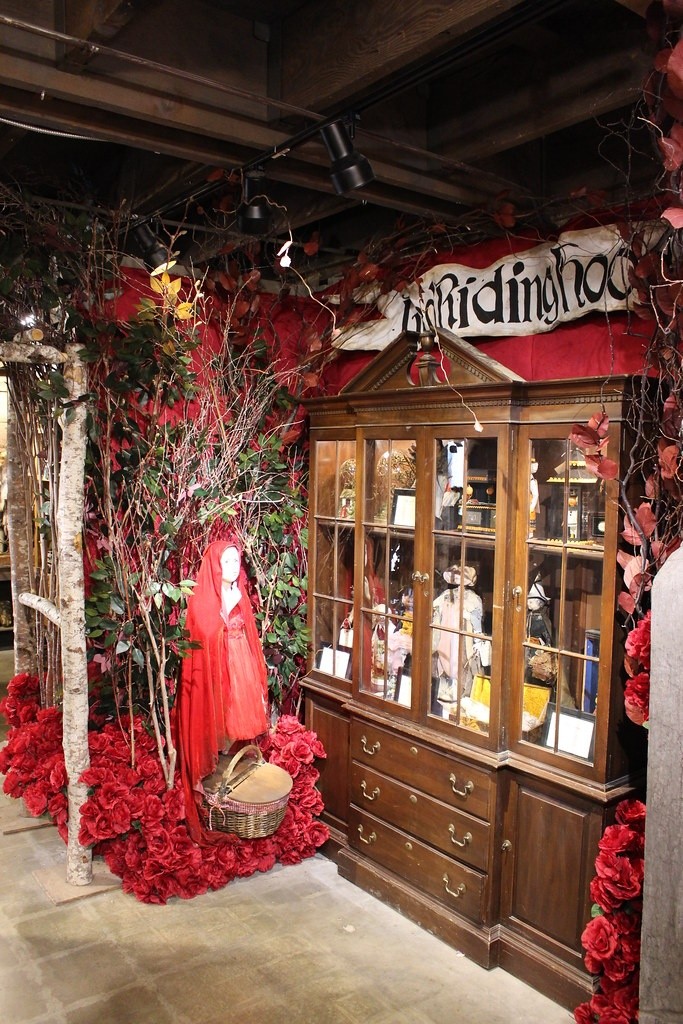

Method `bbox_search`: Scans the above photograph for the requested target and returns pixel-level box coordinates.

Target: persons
[568,489,578,539]
[524,571,553,687]
[178,541,268,784]
[458,487,473,529]
[529,458,540,539]
[341,537,397,693]
[434,565,484,708]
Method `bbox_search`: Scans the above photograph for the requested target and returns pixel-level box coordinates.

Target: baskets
[198,744,293,839]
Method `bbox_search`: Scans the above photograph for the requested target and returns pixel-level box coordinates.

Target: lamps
[320,120,376,197]
[16,286,38,328]
[131,223,175,275]
[236,178,270,237]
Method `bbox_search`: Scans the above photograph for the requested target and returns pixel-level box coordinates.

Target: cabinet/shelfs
[299,370,673,1011]
